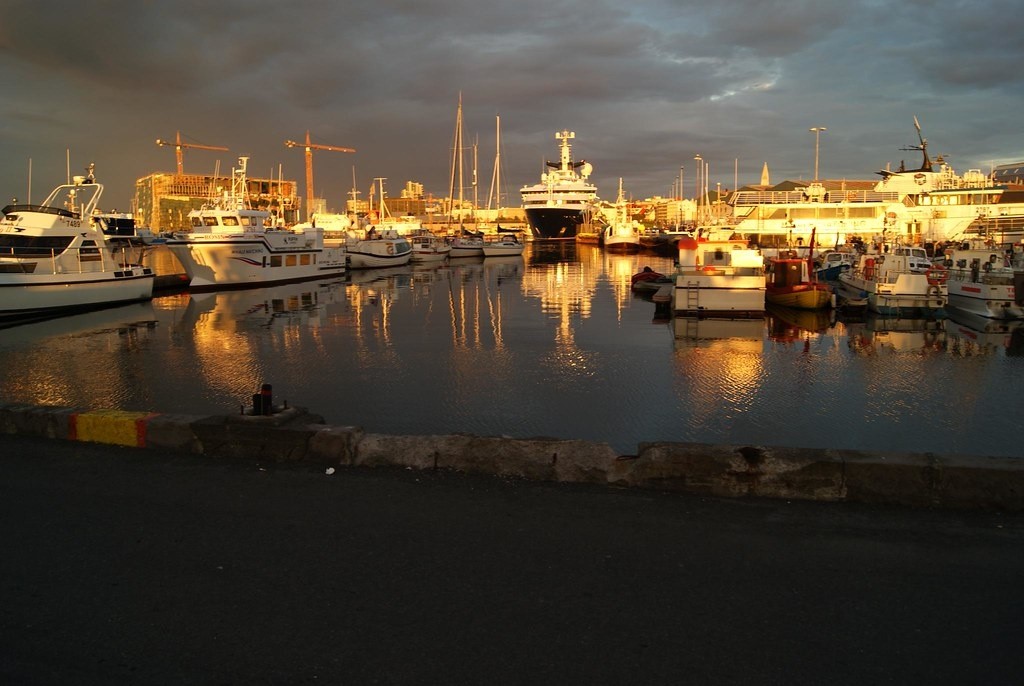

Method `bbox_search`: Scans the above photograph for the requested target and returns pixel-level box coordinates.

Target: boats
[600,177,641,251]
[339,178,413,269]
[444,91,525,257]
[817,227,1024,314]
[164,156,346,286]
[408,225,451,263]
[0,144,156,316]
[764,226,834,310]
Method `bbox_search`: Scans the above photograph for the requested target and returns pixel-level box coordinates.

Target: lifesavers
[926,264,947,285]
[850,191,858,200]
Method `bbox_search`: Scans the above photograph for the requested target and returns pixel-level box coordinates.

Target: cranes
[285,128,356,220]
[155,127,230,174]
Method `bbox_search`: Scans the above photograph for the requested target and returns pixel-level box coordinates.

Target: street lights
[809,127,828,180]
[693,153,709,205]
[667,166,685,200]
[715,182,725,200]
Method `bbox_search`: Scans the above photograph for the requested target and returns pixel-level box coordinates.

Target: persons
[680,224,685,231]
[851,236,863,245]
[688,225,691,228]
[367,227,375,235]
[1004,249,1011,266]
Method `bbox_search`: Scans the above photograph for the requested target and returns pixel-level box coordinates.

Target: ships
[519,129,599,240]
